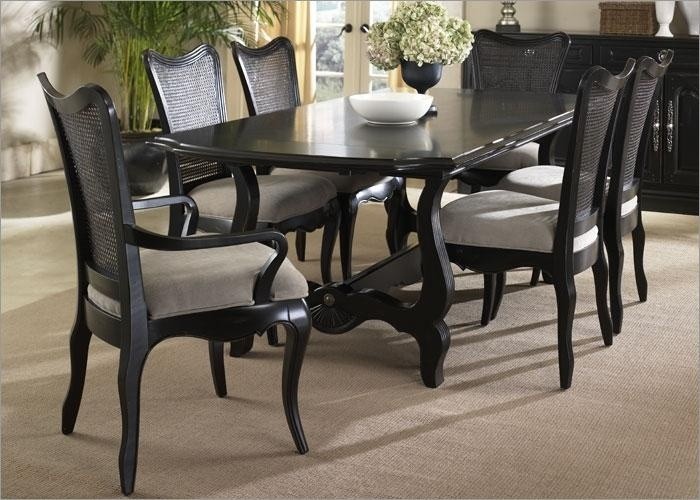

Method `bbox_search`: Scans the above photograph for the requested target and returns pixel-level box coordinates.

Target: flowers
[361,1,475,72]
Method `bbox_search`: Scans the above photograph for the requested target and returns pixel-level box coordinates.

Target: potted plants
[24,1,291,193]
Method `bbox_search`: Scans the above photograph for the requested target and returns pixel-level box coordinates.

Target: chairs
[230,37,405,262]
[36,72,314,498]
[436,57,638,392]
[143,42,339,348]
[459,29,574,193]
[499,48,675,336]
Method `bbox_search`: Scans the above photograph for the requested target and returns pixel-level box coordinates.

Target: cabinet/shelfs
[461,30,699,216]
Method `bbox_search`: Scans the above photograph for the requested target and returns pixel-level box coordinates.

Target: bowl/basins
[348,94,434,126]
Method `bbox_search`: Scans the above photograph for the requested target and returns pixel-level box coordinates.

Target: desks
[144,85,580,390]
